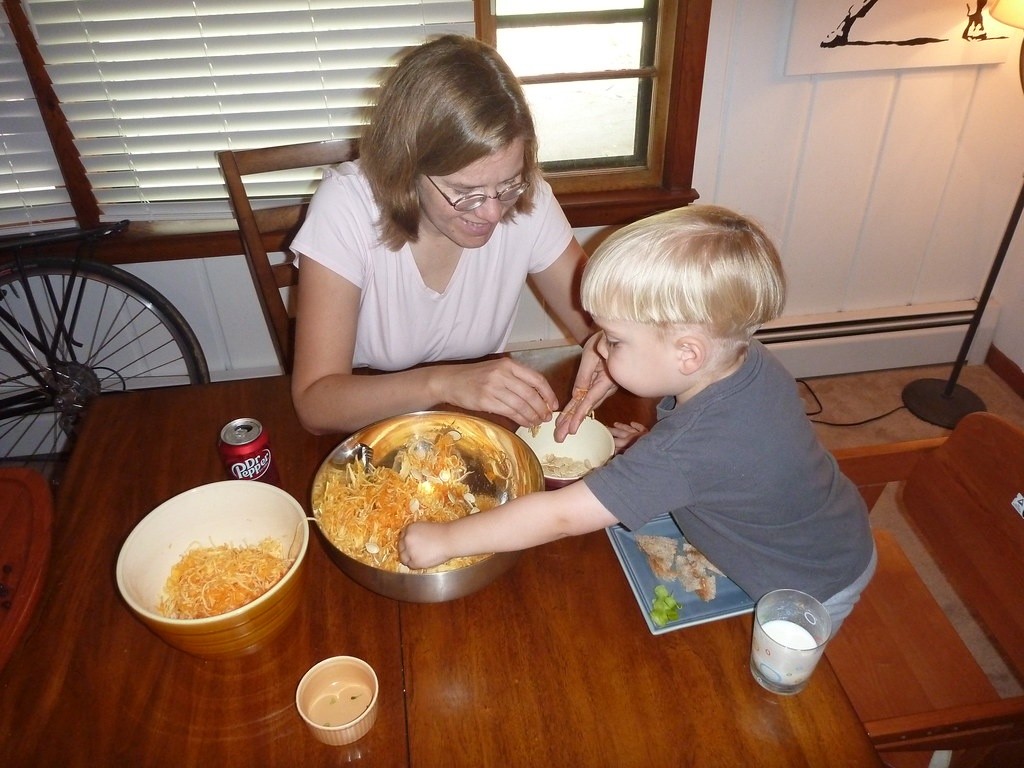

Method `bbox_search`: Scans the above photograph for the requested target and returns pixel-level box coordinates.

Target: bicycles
[0,218,211,464]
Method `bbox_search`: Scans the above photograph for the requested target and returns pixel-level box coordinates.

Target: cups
[749,588,832,695]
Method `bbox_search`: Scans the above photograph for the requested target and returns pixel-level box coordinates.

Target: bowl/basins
[296,656,378,746]
[116,480,310,660]
[515,412,615,491]
[310,410,544,603]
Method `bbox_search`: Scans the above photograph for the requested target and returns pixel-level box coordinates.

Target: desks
[0,344,880,767]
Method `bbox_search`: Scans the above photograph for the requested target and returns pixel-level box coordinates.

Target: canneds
[218,417,282,488]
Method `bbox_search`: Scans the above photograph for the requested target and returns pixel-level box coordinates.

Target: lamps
[895,0,1024,426]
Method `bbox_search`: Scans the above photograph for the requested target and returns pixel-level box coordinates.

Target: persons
[289,32,617,442]
[397,205,878,646]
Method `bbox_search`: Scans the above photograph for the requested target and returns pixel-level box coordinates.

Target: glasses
[426,175,530,211]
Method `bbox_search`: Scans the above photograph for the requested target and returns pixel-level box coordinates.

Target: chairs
[825,412,1024,751]
[213,137,362,372]
